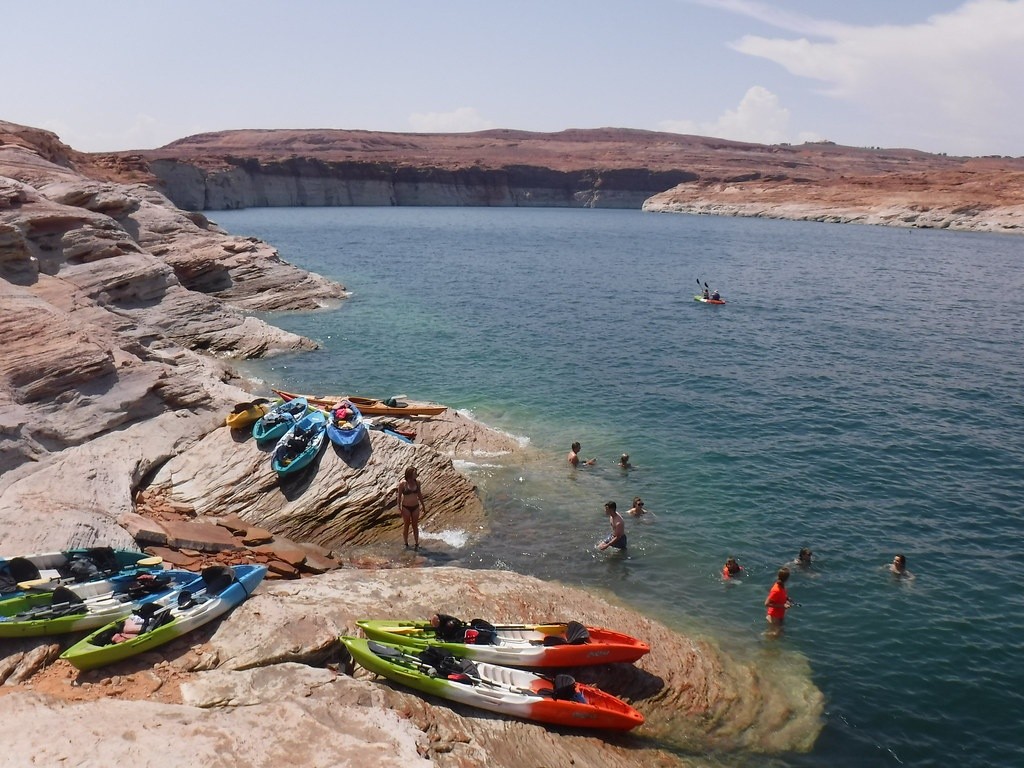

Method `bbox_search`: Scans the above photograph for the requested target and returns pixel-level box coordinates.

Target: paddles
[367,641,538,697]
[11,578,157,621]
[696,278,703,291]
[704,282,713,293]
[360,393,407,404]
[378,622,568,635]
[17,555,163,590]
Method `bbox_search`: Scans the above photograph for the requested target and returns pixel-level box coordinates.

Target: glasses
[805,552,812,557]
[636,503,644,506]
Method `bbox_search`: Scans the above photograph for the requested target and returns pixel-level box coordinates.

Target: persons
[617,453,631,468]
[398,466,426,549]
[711,290,720,300]
[765,567,797,621]
[721,557,744,575]
[598,501,627,551]
[793,547,813,565]
[701,289,709,299]
[626,497,647,514]
[884,554,916,581]
[568,442,597,466]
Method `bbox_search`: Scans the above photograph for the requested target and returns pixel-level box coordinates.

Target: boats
[58,561,272,674]
[339,634,646,735]
[225,397,285,430]
[326,396,367,451]
[355,613,652,670]
[269,410,327,480]
[0,566,200,639]
[270,388,417,444]
[694,294,726,306]
[278,389,449,417]
[0,543,163,601]
[251,394,309,444]
